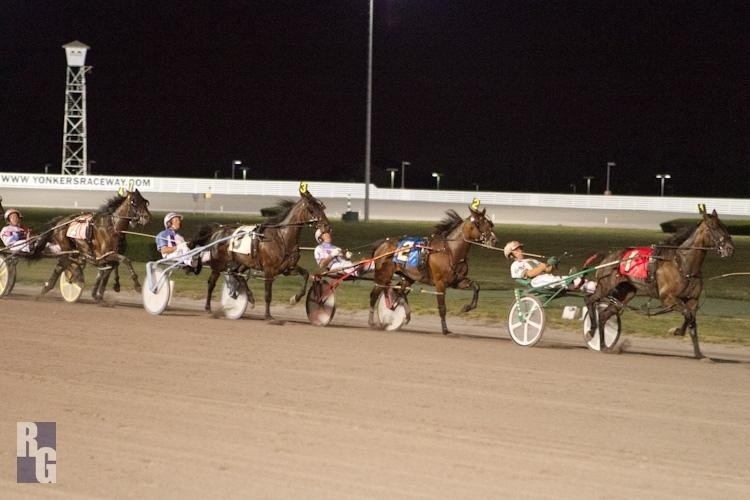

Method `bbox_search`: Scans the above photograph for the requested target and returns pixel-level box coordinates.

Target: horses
[353,205,498,337]
[582,203,736,363]
[26,188,153,306]
[183,182,333,324]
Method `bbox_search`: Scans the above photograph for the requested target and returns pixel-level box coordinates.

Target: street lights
[239,167,250,180]
[232,160,242,179]
[570,184,577,194]
[401,161,411,188]
[606,161,616,195]
[45,163,53,174]
[214,169,220,179]
[582,176,595,194]
[432,173,440,189]
[656,174,671,196]
[385,168,399,187]
[87,160,96,175]
[472,183,479,190]
[72,168,83,175]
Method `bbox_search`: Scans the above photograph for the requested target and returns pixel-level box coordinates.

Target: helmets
[163,212,184,230]
[4,208,24,225]
[315,228,333,244]
[503,240,525,261]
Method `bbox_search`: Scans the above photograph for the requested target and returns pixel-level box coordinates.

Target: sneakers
[190,244,201,269]
[27,231,36,256]
[567,266,577,291]
[357,257,366,277]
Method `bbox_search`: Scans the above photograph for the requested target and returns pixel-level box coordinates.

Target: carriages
[0,188,152,308]
[306,206,499,337]
[503,208,735,364]
[141,188,335,323]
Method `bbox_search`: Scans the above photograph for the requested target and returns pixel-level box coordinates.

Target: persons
[504,240,597,292]
[1,209,59,257]
[157,212,212,268]
[314,228,374,275]
